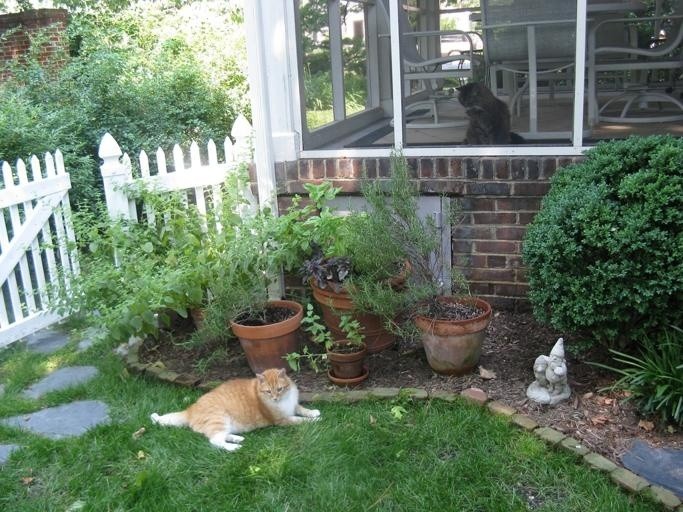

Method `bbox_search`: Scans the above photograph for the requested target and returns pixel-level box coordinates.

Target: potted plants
[284,297,370,385]
[347,143,492,374]
[218,232,304,376]
[274,179,411,353]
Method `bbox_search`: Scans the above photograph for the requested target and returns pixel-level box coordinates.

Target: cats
[455,80,526,146]
[150,367,322,452]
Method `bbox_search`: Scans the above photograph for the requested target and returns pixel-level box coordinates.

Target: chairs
[585,3,683,129]
[377,2,485,125]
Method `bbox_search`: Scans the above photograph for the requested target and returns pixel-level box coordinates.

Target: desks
[484,18,595,140]
[469,1,652,139]
